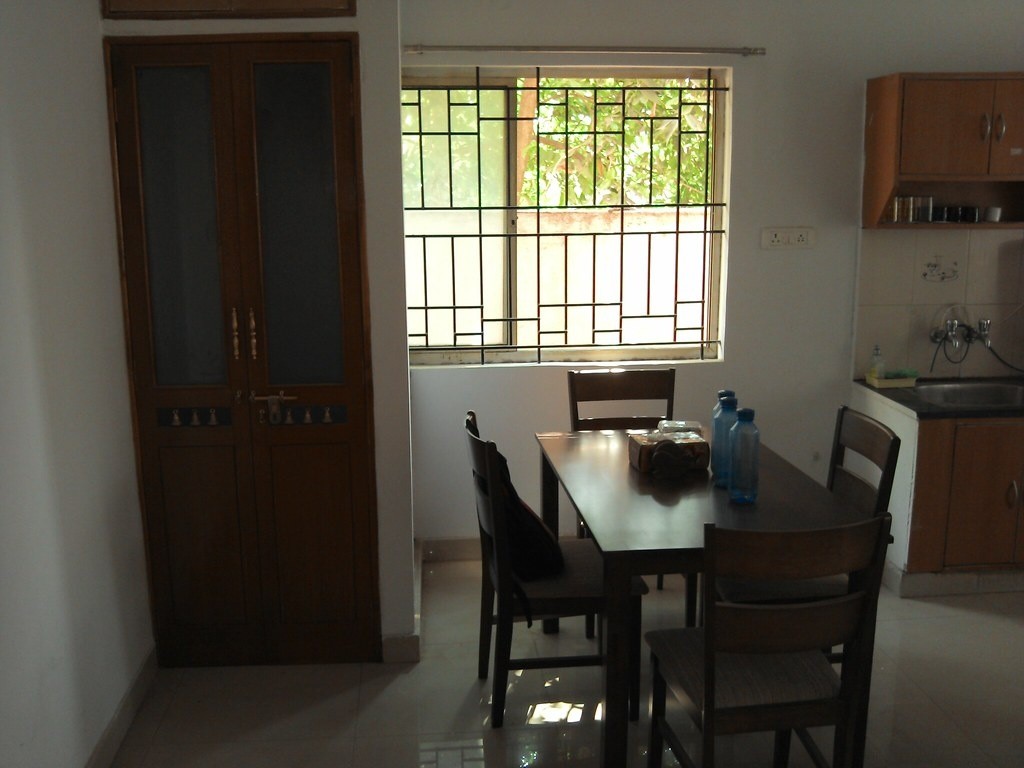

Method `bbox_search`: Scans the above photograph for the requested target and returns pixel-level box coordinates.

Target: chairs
[825,405,901,513]
[566,368,676,588]
[646,513,893,768]
[463,410,649,730]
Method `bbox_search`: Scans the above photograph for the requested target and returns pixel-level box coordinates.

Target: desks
[532,423,869,768]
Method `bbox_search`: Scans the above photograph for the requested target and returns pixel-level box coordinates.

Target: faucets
[976,319,992,348]
[942,319,959,348]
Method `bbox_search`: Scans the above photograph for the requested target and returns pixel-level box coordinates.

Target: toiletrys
[866,345,885,379]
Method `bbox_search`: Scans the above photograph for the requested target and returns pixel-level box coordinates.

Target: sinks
[915,384,1024,407]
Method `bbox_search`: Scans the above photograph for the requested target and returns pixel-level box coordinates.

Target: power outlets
[761,227,789,250]
[787,226,814,250]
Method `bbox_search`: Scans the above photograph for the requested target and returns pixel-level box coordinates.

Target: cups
[890,195,1003,226]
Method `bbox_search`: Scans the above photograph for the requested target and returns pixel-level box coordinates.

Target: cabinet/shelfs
[94,28,384,666]
[848,392,1024,575]
[862,70,1024,229]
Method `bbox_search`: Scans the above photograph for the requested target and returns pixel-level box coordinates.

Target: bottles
[712,397,742,490]
[868,344,885,380]
[726,408,761,505]
[713,390,738,439]
